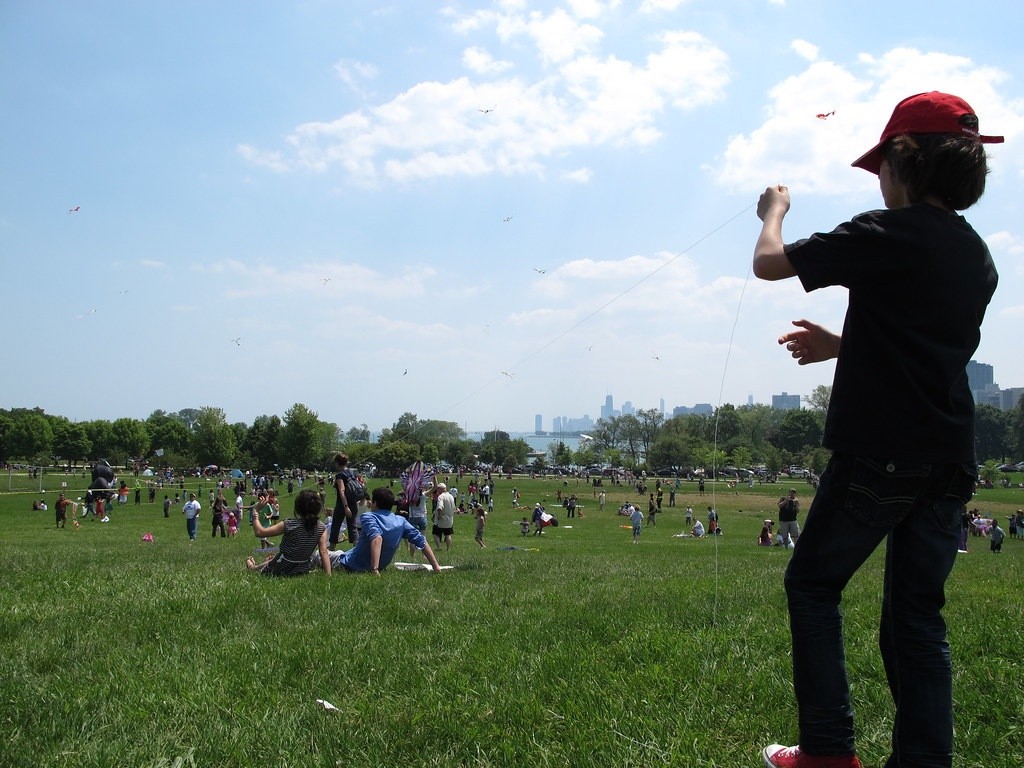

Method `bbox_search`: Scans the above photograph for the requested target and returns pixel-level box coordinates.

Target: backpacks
[341,468,365,503]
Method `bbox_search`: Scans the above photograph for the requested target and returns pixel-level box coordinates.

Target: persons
[65,465,87,478]
[198,479,279,550]
[752,91,1005,768]
[0,460,29,470]
[33,488,113,529]
[239,465,306,498]
[182,493,201,541]
[28,467,37,479]
[189,465,233,481]
[163,495,170,518]
[313,452,441,577]
[132,453,187,506]
[246,489,331,576]
[959,475,1024,553]
[432,465,819,549]
[108,476,129,504]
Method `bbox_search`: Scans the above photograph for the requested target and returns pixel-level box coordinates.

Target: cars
[995,461,1024,472]
[553,466,572,476]
[603,469,624,477]
[656,462,809,477]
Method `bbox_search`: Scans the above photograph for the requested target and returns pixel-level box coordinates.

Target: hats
[229,512,234,517]
[1016,509,1024,514]
[240,492,246,495]
[789,488,797,493]
[438,483,446,488]
[191,493,196,498]
[977,515,981,518]
[535,503,540,508]
[851,90,1004,175]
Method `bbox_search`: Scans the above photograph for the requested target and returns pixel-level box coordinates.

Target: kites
[816,112,835,119]
[400,461,435,504]
[61,500,85,529]
[579,434,593,442]
[204,465,218,472]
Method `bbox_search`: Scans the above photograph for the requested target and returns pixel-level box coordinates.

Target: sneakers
[763,744,861,768]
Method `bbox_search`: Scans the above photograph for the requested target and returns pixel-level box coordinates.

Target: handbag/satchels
[777,532,795,548]
[517,492,520,498]
[541,520,551,527]
[540,513,553,522]
[549,517,558,526]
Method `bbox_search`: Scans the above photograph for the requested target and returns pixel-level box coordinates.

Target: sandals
[246,556,254,568]
